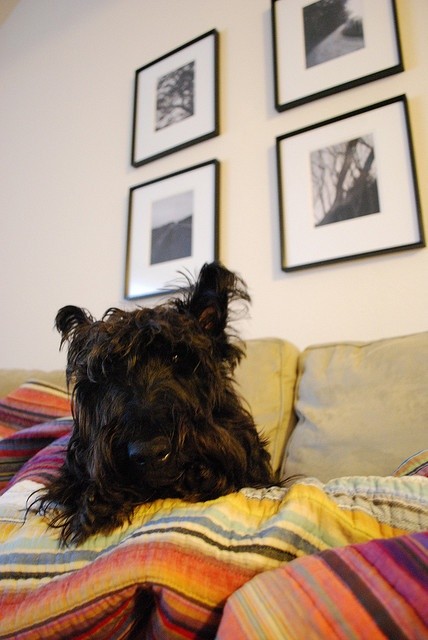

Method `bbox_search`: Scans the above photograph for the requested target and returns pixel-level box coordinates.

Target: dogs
[17,262,307,550]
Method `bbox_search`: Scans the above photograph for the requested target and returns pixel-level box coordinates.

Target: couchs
[1,330,427,486]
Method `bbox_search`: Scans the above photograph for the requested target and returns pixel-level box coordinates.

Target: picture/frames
[123,160,219,301]
[270,0,404,113]
[129,28,219,166]
[275,94,425,273]
